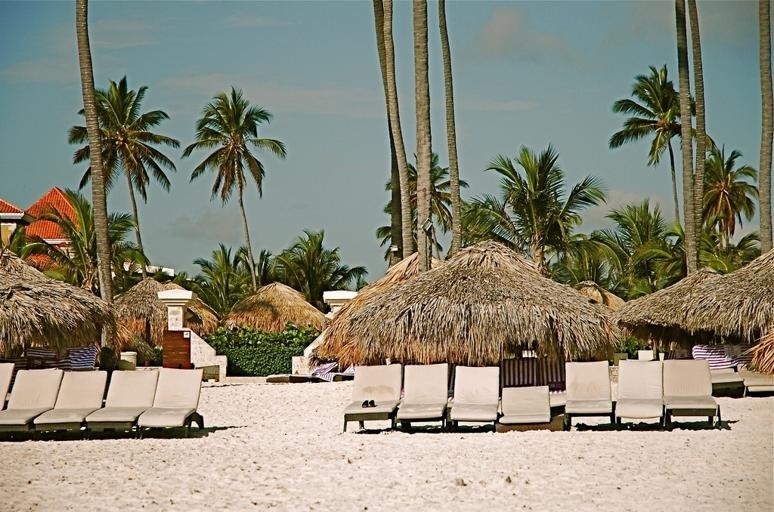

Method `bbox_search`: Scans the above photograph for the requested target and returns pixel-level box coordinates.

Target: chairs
[451,356,565,429]
[726,344,774,396]
[663,360,720,427]
[615,360,664,428]
[343,364,402,432]
[397,364,450,430]
[694,344,743,394]
[0,345,204,437]
[563,360,615,430]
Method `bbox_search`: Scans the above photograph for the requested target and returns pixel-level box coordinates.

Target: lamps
[421,219,434,233]
[389,245,399,252]
